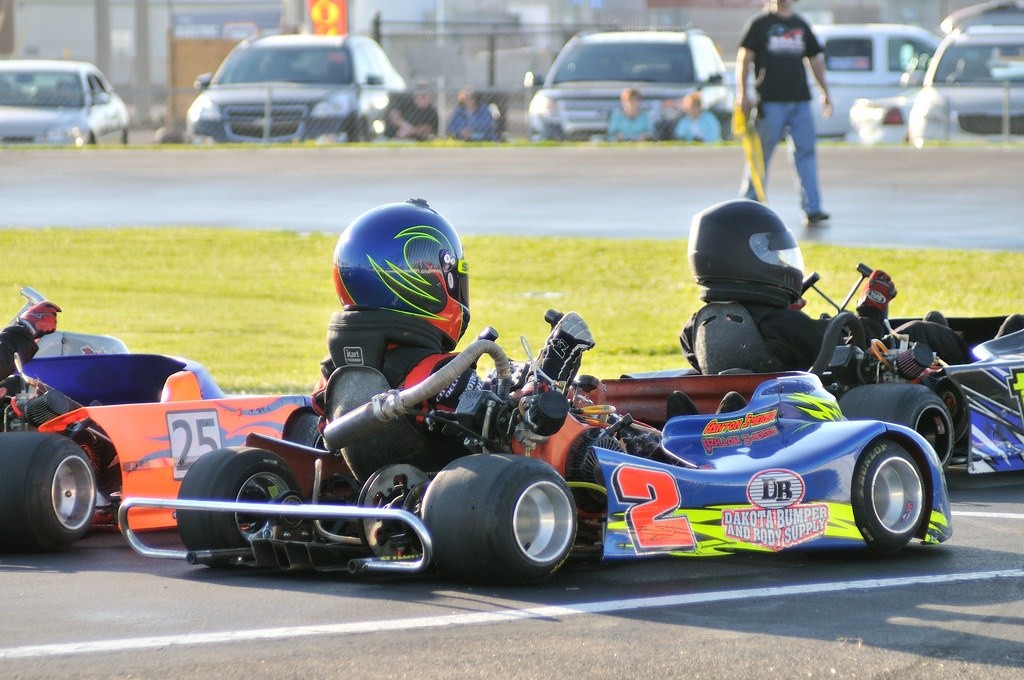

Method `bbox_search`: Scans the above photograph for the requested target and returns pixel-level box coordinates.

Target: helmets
[687,197,804,308]
[331,196,470,350]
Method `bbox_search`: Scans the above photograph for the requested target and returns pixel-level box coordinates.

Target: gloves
[524,310,595,396]
[17,300,62,340]
[855,268,898,319]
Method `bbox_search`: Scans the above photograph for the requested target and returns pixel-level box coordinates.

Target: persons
[384,81,502,143]
[607,86,725,141]
[737,1,831,222]
[311,198,593,484]
[680,200,969,400]
[0,302,61,400]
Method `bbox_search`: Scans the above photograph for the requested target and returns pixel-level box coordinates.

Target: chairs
[317,365,452,467]
[691,302,781,378]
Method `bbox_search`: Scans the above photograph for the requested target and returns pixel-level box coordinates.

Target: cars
[908,0,1023,147]
[0,57,130,147]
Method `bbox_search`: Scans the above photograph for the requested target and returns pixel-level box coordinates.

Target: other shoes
[994,313,1024,339]
[714,391,748,414]
[665,390,699,424]
[922,310,949,328]
[807,211,828,223]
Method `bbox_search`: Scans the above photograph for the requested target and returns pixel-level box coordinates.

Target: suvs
[184,30,408,146]
[520,25,736,142]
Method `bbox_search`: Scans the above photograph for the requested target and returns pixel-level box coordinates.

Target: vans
[807,24,950,140]
